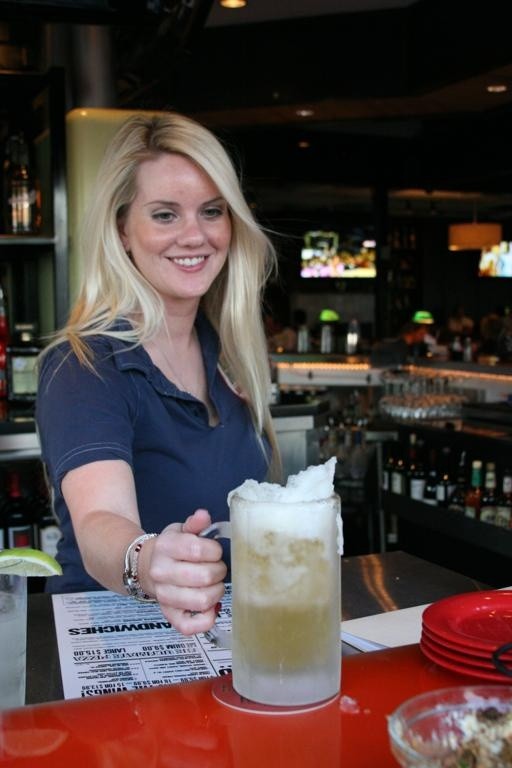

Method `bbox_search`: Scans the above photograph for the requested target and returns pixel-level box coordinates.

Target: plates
[418,587,511,686]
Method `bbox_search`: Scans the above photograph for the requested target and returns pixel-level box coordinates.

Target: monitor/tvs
[297,220,381,282]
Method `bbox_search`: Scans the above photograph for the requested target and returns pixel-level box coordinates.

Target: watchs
[122,533,157,603]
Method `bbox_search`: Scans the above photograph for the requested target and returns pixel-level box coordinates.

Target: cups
[188,488,347,708]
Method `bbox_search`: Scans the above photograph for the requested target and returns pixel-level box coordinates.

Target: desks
[0,637,487,767]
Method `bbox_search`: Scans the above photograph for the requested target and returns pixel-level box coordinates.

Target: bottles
[381,430,511,529]
[297,323,332,353]
[6,308,47,416]
[0,287,7,420]
[449,335,473,363]
[4,118,42,237]
[323,418,366,481]
[345,317,359,358]
[341,389,368,427]
[0,469,59,580]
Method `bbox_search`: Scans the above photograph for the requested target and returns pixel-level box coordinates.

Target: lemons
[0,547,66,580]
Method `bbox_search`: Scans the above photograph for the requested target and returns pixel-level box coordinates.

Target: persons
[261,303,512,367]
[30,109,283,638]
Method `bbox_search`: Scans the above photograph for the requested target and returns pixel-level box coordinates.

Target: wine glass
[374,372,465,423]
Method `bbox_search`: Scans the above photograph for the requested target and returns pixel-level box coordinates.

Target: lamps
[446,221,501,254]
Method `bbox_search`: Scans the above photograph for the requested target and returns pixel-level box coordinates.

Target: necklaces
[145,333,206,400]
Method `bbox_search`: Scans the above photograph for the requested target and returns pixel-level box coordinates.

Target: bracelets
[131,531,159,601]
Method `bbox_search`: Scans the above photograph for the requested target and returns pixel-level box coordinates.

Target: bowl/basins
[386,686,511,766]
